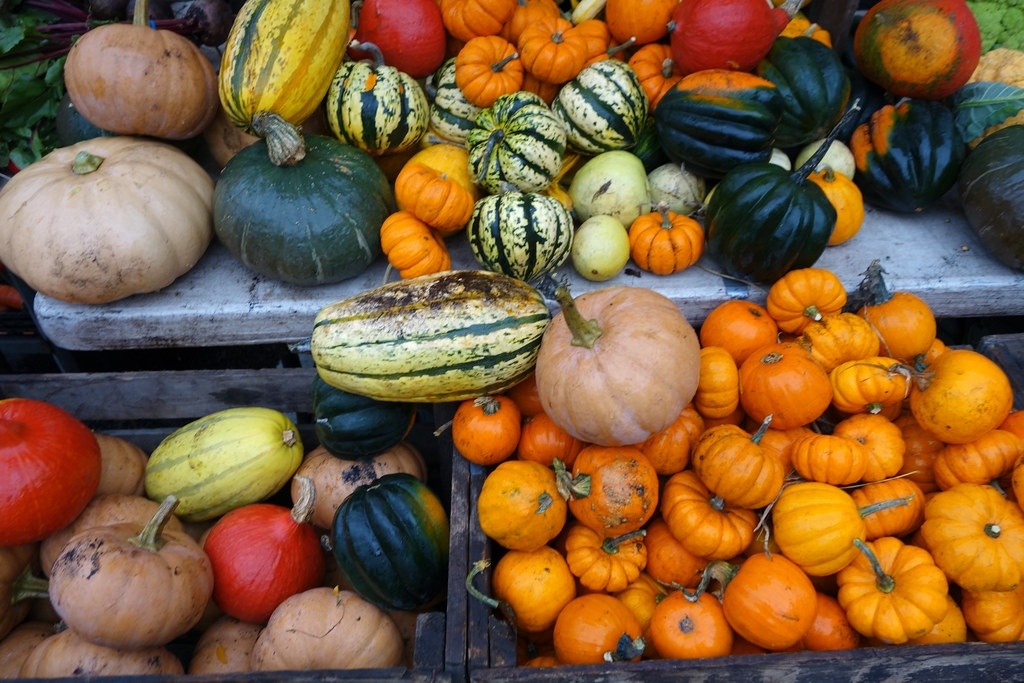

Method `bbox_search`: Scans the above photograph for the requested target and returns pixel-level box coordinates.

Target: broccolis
[950,47,1024,149]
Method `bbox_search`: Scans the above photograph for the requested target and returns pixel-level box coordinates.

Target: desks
[34,206,1024,368]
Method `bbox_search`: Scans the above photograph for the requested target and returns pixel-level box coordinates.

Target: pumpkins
[0,0,1024,680]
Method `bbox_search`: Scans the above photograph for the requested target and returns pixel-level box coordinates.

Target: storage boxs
[0,367,471,683]
[469,332,1024,683]
